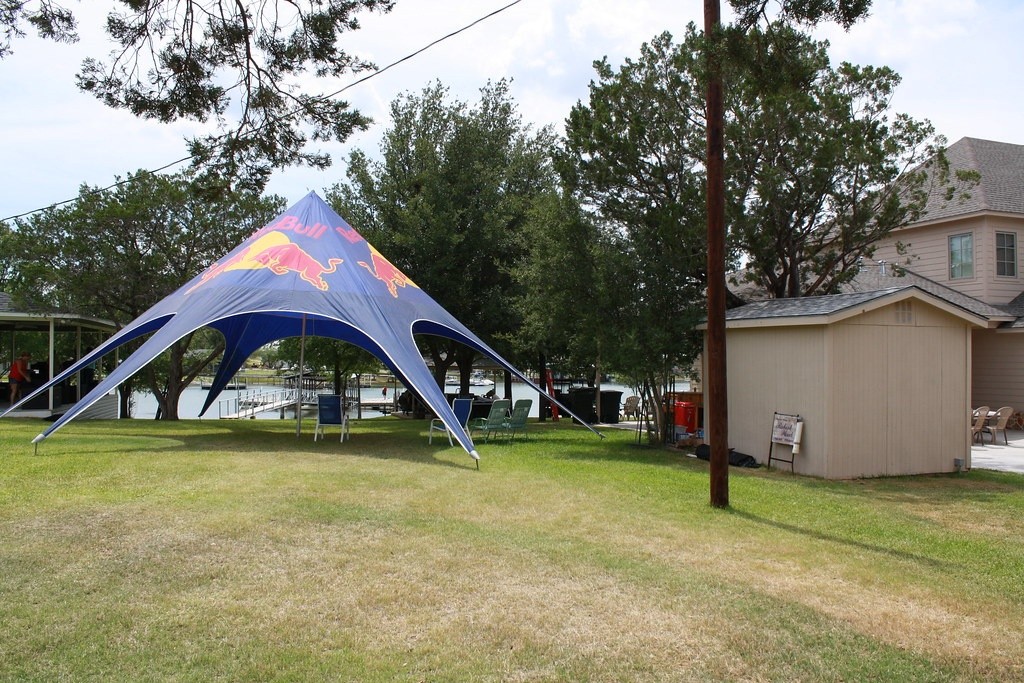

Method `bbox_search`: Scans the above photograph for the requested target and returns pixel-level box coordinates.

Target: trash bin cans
[567,387,599,425]
[674,401,697,433]
[600,390,624,424]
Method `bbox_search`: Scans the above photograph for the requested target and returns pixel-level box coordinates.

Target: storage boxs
[18,381,63,409]
[668,424,704,447]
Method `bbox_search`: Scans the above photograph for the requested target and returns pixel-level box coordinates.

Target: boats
[474,380,489,386]
[446,372,494,386]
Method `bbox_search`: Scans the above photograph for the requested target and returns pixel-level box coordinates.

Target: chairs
[971,405,990,446]
[471,400,512,445]
[618,396,640,420]
[493,399,532,443]
[429,397,475,448]
[978,407,1014,445]
[314,394,349,443]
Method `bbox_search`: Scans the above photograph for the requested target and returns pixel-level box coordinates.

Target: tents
[0,190,608,469]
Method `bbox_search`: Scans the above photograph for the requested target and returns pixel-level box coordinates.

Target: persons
[8,352,32,408]
[398,393,410,416]
[81,347,96,393]
[382,385,387,400]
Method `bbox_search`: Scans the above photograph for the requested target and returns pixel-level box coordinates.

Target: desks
[973,409,1001,435]
